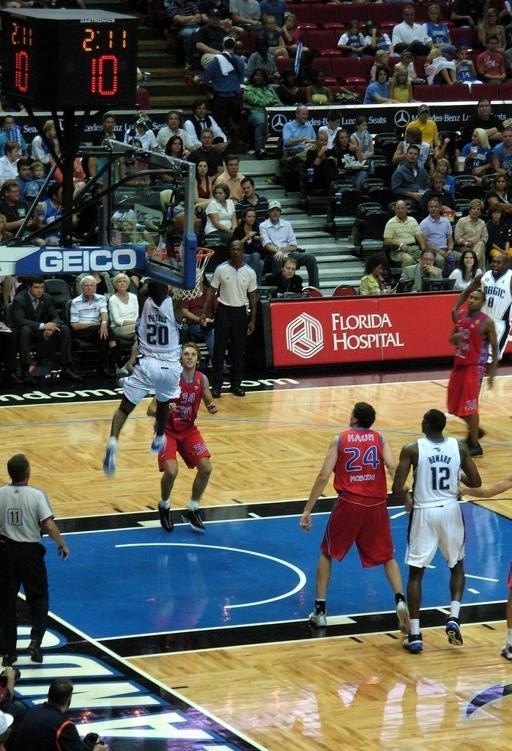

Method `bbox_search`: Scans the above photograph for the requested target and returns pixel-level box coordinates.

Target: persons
[460,127,494,174]
[125,112,158,151]
[194,159,215,203]
[190,9,231,70]
[71,276,127,384]
[391,128,434,170]
[151,136,189,196]
[368,49,390,86]
[188,128,225,177]
[0,455,69,667]
[258,14,286,60]
[110,273,139,339]
[460,473,511,661]
[1,142,20,179]
[234,177,271,225]
[395,49,424,83]
[1,667,29,721]
[274,72,308,105]
[147,342,219,531]
[27,120,57,172]
[183,99,230,150]
[282,105,317,168]
[484,0,512,26]
[450,0,484,28]
[488,206,512,259]
[337,20,367,58]
[435,131,460,171]
[306,133,339,188]
[419,198,462,257]
[233,40,249,64]
[259,201,319,293]
[451,254,512,374]
[244,38,280,84]
[264,257,303,298]
[92,115,124,148]
[436,159,460,198]
[301,402,411,634]
[475,35,511,83]
[449,250,484,291]
[457,46,485,84]
[118,138,151,187]
[32,185,79,247]
[309,69,334,104]
[364,67,400,103]
[455,199,488,272]
[483,174,512,221]
[317,112,342,147]
[12,277,71,381]
[393,4,437,56]
[1,710,14,750]
[384,201,446,272]
[360,255,391,295]
[386,65,420,102]
[194,37,244,151]
[90,156,119,202]
[0,680,107,750]
[103,279,185,477]
[156,111,197,151]
[350,117,374,176]
[422,5,464,84]
[392,146,434,199]
[400,250,442,292]
[229,0,262,35]
[405,105,441,158]
[204,183,238,244]
[231,209,264,279]
[462,97,506,151]
[214,157,245,203]
[163,0,209,42]
[181,272,217,368]
[421,175,456,222]
[327,78,354,96]
[477,8,507,51]
[245,69,286,159]
[197,0,245,35]
[493,126,512,175]
[14,158,37,195]
[24,163,48,198]
[1,180,42,240]
[392,409,482,652]
[200,241,258,398]
[448,290,498,456]
[51,158,86,185]
[332,129,357,180]
[365,20,394,55]
[1,116,27,159]
[278,12,312,58]
[258,0,290,31]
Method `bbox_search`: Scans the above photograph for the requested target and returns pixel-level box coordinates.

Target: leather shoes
[21,367,32,379]
[61,369,81,383]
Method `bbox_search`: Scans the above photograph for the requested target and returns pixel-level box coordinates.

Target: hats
[268,201,281,211]
[417,105,430,113]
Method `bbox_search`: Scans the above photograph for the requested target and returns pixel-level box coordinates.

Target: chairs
[275,135,304,195]
[201,244,232,285]
[333,283,356,296]
[298,156,330,201]
[64,298,103,374]
[422,3,453,26]
[389,201,414,221]
[453,174,477,197]
[359,179,387,200]
[446,26,477,54]
[341,3,366,29]
[328,177,359,228]
[432,131,460,158]
[43,279,70,319]
[313,3,342,29]
[287,4,317,29]
[307,55,338,99]
[354,203,386,256]
[373,133,400,159]
[301,27,341,56]
[471,83,497,99]
[232,0,511,103]
[360,54,377,80]
[363,155,389,177]
[301,286,322,297]
[410,80,444,103]
[497,82,511,98]
[482,173,501,198]
[441,83,470,100]
[384,54,402,78]
[271,56,298,86]
[453,198,473,223]
[395,3,420,24]
[330,52,367,96]
[367,3,396,31]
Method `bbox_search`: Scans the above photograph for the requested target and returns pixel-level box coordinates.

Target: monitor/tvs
[398,277,416,293]
[254,287,277,300]
[285,292,309,302]
[420,278,455,292]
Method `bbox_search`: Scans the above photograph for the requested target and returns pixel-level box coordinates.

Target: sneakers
[403,631,423,653]
[308,612,327,625]
[230,383,245,396]
[1,653,18,666]
[150,434,167,453]
[501,644,512,660]
[180,508,205,531]
[103,441,117,476]
[465,443,483,457]
[211,388,221,398]
[158,501,173,531]
[476,428,486,438]
[26,639,42,662]
[446,617,463,645]
[396,600,411,633]
